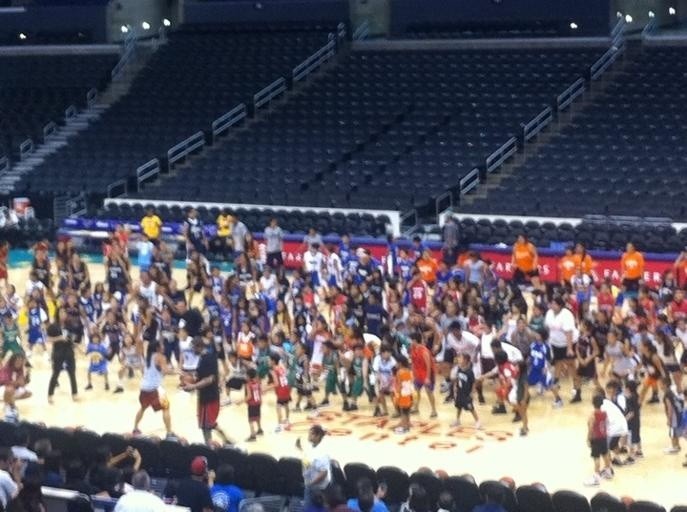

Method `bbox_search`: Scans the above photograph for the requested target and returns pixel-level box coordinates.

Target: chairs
[0,416,687,511]
[1,14,686,268]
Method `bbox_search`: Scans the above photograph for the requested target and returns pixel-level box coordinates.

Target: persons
[1,196,687,510]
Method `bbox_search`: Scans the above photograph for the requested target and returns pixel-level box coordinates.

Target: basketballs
[180,376,194,388]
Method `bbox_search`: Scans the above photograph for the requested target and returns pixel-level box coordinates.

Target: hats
[190,455,208,475]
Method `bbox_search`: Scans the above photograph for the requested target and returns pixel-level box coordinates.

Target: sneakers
[0,372,235,450]
[488,397,685,486]
[240,399,479,443]
